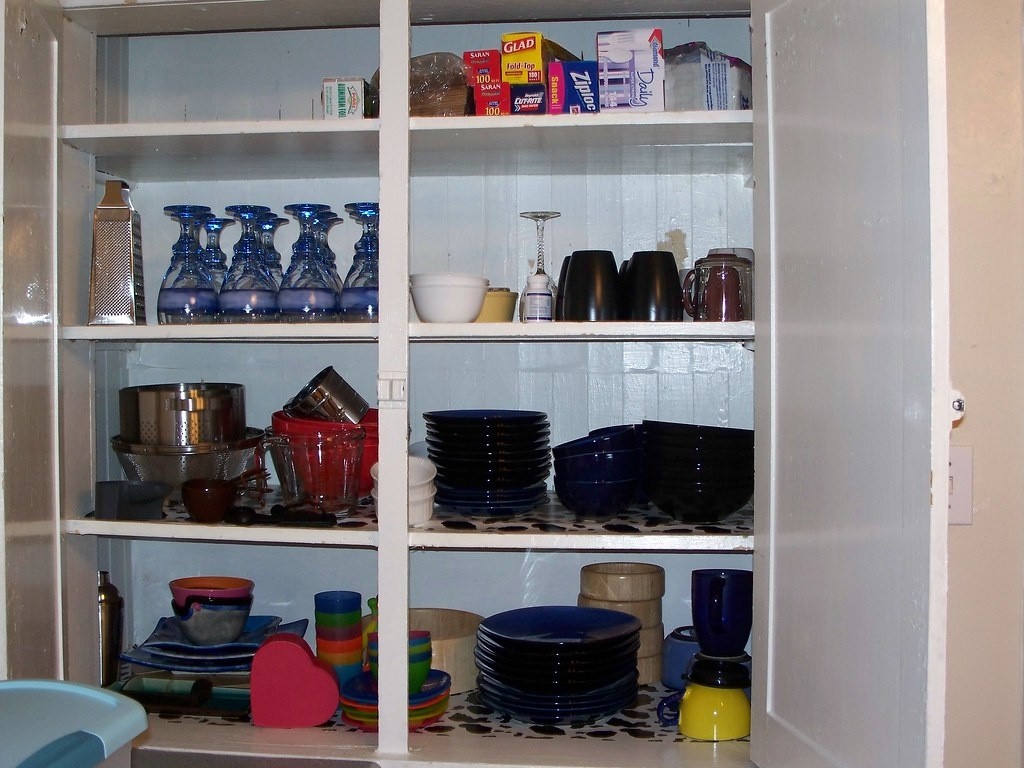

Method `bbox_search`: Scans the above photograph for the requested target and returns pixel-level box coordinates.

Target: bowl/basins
[658,568,756,742]
[551,419,755,525]
[169,576,255,645]
[410,271,518,322]
[313,590,432,695]
[370,456,438,525]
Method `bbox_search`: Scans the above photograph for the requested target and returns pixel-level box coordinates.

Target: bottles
[522,274,553,322]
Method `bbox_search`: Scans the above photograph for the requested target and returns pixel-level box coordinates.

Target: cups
[284,365,369,426]
[554,249,755,323]
[255,426,364,521]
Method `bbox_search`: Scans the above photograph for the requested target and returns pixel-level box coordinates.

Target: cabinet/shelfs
[48,2,769,768]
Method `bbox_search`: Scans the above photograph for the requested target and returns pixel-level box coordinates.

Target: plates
[422,408,553,517]
[576,561,666,685]
[338,668,452,732]
[472,605,642,727]
[121,616,310,716]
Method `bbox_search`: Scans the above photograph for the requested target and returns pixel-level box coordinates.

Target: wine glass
[519,210,561,320]
[156,202,379,324]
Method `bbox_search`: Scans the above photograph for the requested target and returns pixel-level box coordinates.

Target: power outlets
[948,448,973,525]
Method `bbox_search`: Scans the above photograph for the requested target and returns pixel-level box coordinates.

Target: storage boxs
[1,681,149,768]
[320,28,750,123]
